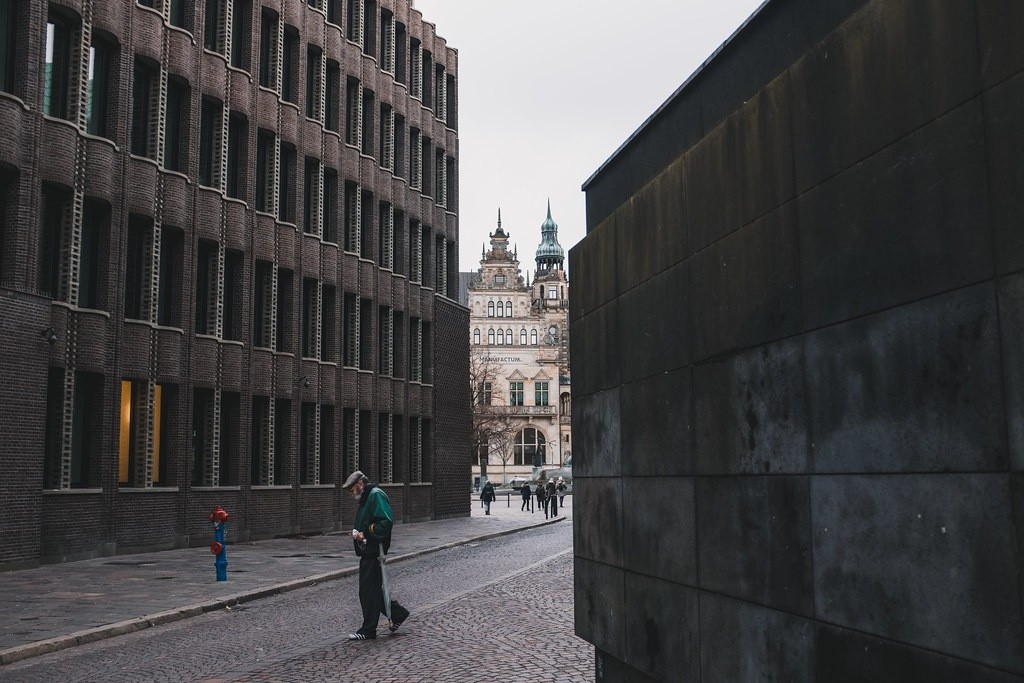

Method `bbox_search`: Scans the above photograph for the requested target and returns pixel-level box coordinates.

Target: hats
[524,480,529,484]
[342,471,365,489]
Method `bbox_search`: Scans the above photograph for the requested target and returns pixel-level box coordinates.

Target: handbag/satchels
[481,500,483,508]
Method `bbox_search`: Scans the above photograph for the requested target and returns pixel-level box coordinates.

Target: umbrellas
[370,523,391,629]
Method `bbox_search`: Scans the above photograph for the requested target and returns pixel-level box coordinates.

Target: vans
[519,467,573,495]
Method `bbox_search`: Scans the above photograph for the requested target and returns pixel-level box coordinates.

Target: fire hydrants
[209,505,229,581]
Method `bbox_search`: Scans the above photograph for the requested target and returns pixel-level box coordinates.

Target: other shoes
[348,633,376,640]
[527,509,530,511]
[389,611,410,632]
[521,508,523,511]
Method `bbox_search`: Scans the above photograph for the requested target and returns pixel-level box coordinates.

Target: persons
[480,481,496,515]
[343,471,410,641]
[520,482,531,511]
[535,476,567,511]
